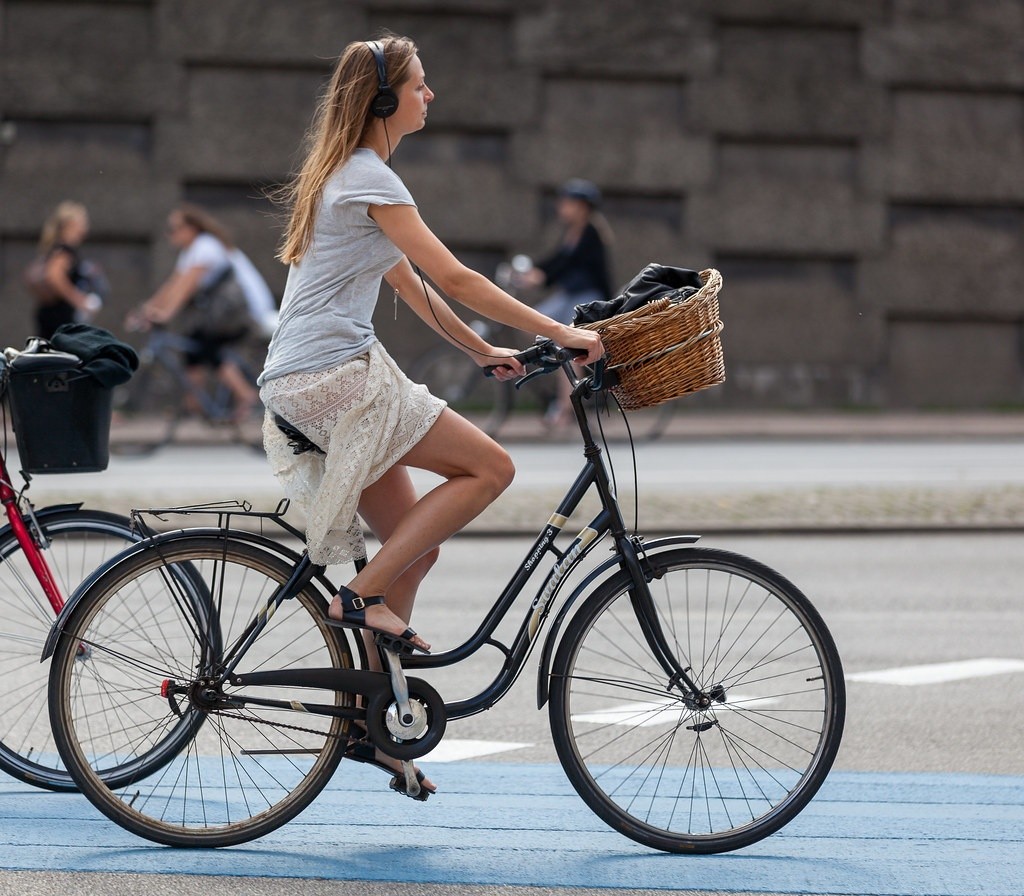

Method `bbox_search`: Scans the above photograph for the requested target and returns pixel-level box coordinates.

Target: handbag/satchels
[176,267,251,345]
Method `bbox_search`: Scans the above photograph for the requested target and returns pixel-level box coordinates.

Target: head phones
[364,41,399,119]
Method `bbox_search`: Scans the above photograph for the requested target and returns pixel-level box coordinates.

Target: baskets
[570,268,726,412]
[10,366,111,474]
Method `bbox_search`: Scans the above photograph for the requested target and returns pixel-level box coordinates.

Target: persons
[500,174,618,428]
[254,37,608,795]
[122,202,281,427]
[21,200,126,425]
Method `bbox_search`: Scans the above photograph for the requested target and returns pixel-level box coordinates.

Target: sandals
[323,586,431,655]
[342,723,436,794]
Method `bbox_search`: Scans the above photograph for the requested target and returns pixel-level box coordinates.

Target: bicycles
[409,268,678,447]
[0,336,223,796]
[106,310,268,463]
[41,268,847,855]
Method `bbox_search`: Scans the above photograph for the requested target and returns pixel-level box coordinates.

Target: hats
[564,179,602,203]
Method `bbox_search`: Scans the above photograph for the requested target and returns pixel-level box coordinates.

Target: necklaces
[393,245,401,321]
